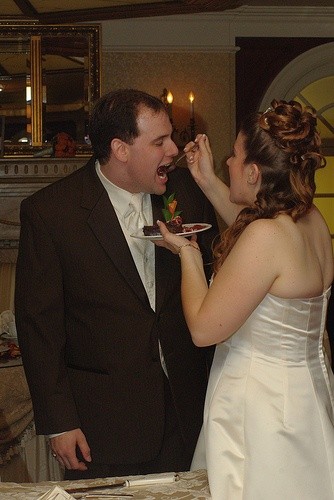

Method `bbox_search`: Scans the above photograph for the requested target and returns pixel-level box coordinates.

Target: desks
[0,469,211,500]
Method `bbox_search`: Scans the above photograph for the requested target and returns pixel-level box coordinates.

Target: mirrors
[0,23,102,158]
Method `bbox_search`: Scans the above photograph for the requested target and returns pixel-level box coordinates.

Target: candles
[189,91,195,118]
[167,89,173,120]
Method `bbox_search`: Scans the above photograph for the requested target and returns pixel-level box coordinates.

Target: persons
[150,99,334,500]
[14,89,222,480]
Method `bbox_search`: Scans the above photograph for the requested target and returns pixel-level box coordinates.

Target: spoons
[158,134,206,175]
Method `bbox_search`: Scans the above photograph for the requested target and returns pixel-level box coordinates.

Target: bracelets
[178,244,201,258]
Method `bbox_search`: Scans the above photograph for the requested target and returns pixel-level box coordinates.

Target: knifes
[65,472,180,498]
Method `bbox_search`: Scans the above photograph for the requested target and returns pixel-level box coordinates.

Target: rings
[52,454,57,457]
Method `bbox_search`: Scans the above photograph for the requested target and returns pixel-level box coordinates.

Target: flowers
[161,192,183,220]
[33,132,76,158]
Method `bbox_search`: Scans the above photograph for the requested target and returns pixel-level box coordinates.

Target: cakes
[142,215,182,235]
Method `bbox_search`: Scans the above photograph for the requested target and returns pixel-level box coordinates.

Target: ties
[124,192,148,255]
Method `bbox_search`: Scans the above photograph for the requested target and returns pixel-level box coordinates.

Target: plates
[130,224,212,241]
